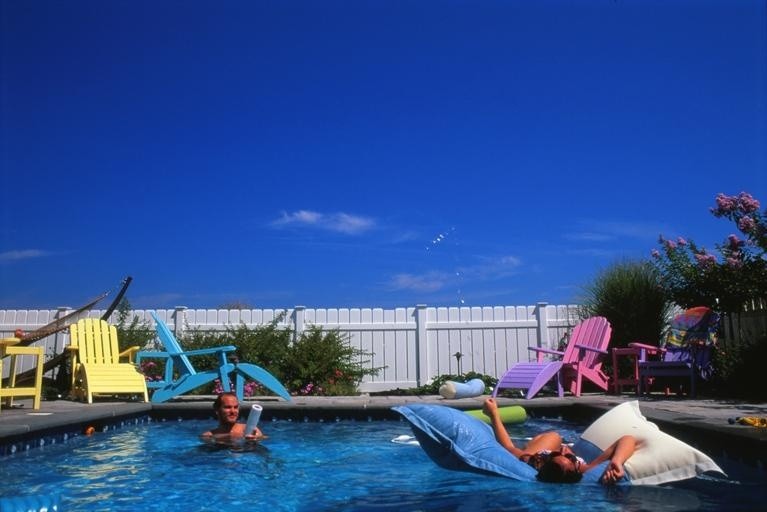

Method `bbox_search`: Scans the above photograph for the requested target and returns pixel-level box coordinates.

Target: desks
[0,338,45,410]
[610,348,657,394]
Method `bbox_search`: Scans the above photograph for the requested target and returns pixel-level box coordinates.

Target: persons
[482,398,636,485]
[201,392,262,438]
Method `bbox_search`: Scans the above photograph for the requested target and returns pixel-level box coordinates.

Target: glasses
[549,452,577,464]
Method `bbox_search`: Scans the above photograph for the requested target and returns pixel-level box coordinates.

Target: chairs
[490,316,613,399]
[136,309,291,403]
[628,306,722,399]
[66,317,151,403]
